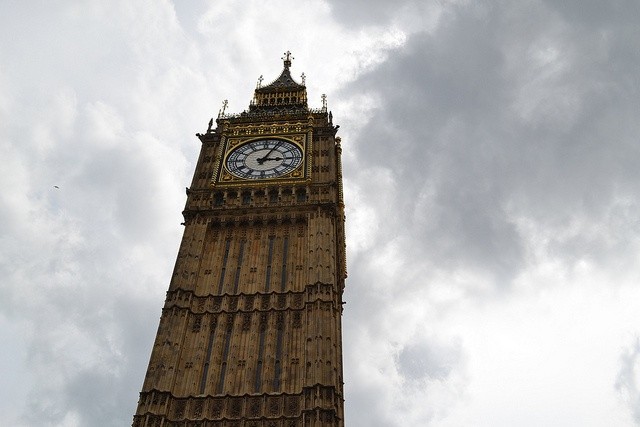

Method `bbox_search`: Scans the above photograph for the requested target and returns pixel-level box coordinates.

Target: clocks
[223,136,303,180]
